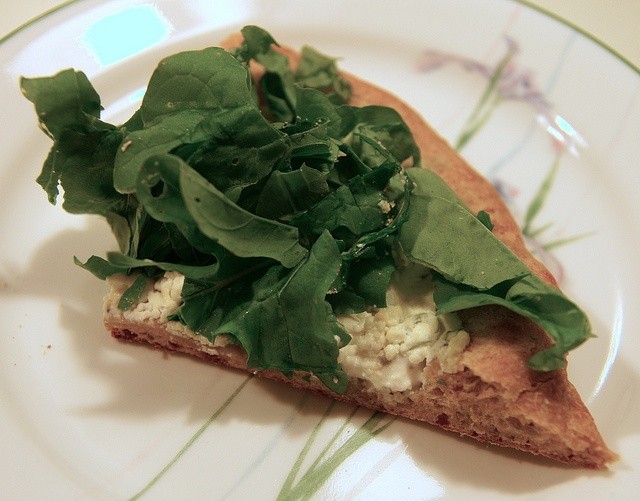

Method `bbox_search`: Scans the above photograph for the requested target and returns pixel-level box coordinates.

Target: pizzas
[19,24,619,472]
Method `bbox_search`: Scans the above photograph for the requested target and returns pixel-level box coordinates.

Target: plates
[1,2,640,500]
